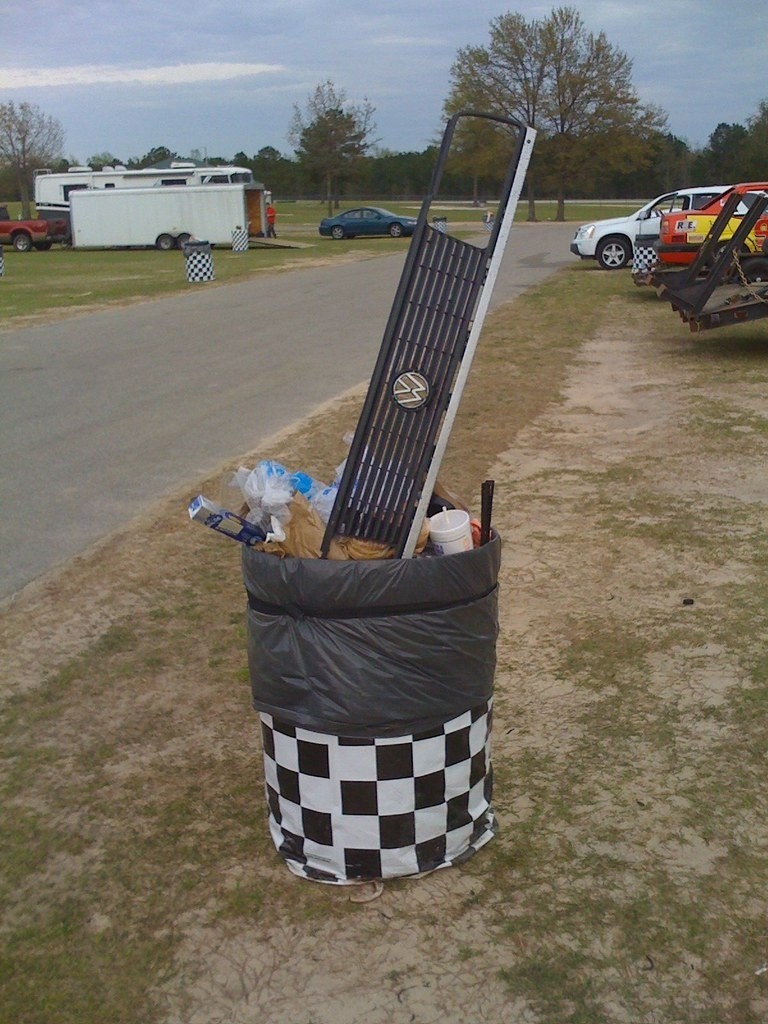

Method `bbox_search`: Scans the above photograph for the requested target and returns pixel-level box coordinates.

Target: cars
[570,185,768,270]
[653,182,768,267]
[319,207,417,240]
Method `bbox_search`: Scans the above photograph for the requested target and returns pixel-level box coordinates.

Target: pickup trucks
[0,207,67,252]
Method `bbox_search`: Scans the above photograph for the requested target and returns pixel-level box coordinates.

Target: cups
[429,508,475,554]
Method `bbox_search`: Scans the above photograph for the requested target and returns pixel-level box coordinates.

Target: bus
[33,162,253,250]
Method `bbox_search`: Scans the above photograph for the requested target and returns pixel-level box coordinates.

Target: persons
[266,202,277,239]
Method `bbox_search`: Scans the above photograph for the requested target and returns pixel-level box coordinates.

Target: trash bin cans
[631,240,661,275]
[238,488,506,887]
[479,200,486,208]
[0,245,6,277]
[230,229,249,252]
[433,216,448,234]
[180,240,216,283]
[482,214,495,232]
